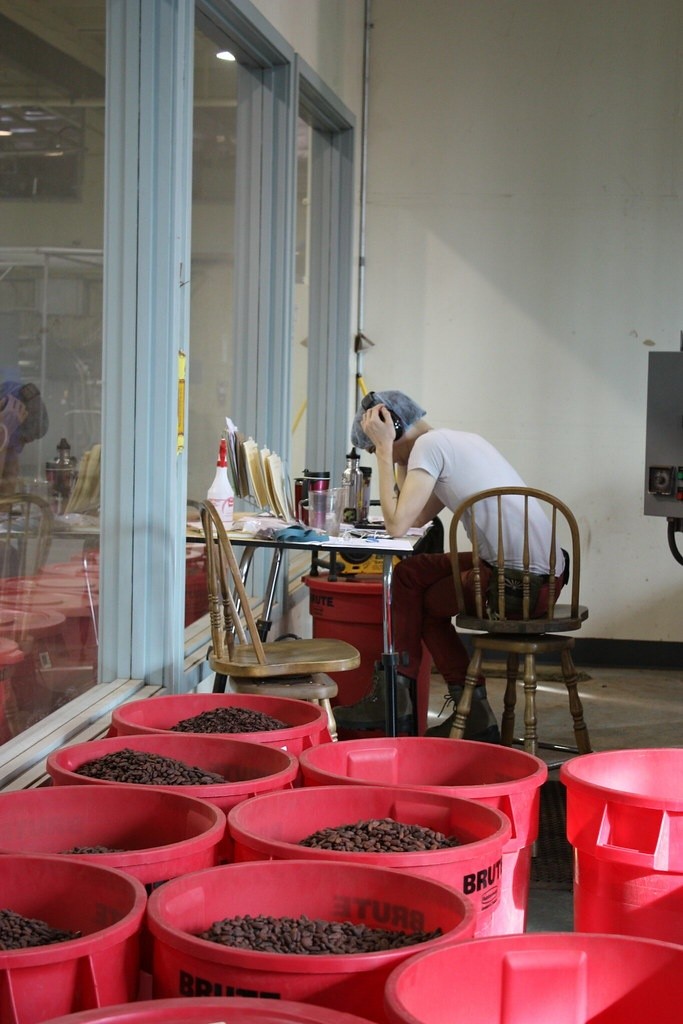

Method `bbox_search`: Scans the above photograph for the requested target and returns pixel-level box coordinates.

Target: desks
[185,505,434,738]
[0,496,100,574]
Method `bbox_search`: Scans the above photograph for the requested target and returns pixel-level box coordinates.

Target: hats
[0,381,49,440]
[351,391,427,454]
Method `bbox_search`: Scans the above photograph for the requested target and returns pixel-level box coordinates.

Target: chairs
[450,486,594,772]
[0,492,55,736]
[198,500,360,742]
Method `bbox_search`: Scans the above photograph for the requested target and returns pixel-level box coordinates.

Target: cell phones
[355,524,385,529]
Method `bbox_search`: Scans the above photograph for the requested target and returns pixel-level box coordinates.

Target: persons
[350,391,568,745]
[0,380,49,579]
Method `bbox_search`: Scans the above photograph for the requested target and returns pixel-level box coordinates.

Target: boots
[424,681,500,744]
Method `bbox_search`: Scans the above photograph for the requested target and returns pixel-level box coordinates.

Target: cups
[303,470,330,528]
[293,477,310,526]
[298,490,345,537]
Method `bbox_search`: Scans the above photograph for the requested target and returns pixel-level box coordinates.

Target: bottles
[341,446,363,523]
[357,465,373,520]
[45,438,78,513]
[204,440,235,531]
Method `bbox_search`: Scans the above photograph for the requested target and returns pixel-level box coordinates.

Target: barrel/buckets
[0,688,683,1024]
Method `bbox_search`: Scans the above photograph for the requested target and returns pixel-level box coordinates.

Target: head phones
[361,392,404,442]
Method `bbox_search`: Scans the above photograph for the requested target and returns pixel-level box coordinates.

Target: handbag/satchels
[487,568,541,615]
